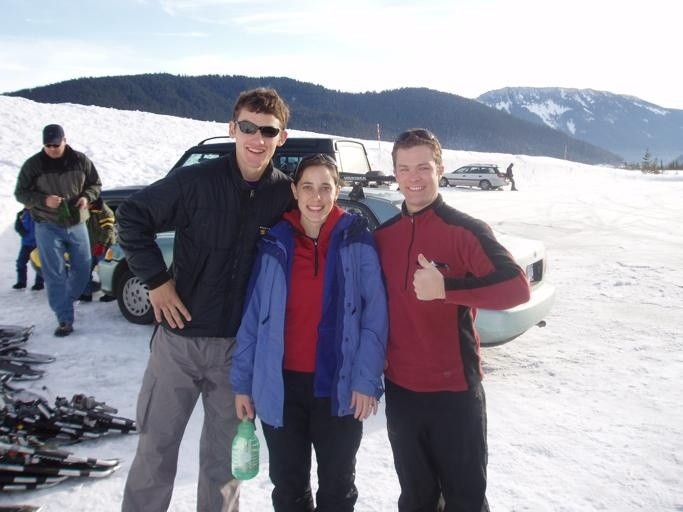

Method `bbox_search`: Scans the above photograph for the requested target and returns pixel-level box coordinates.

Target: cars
[440,163,509,191]
[96,182,555,346]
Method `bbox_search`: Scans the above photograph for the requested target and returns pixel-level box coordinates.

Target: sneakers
[14,282,44,289]
[55,322,73,336]
[99,294,116,302]
[78,294,92,300]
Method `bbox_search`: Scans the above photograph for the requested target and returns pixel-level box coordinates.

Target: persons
[507,163,518,191]
[14,124,103,338]
[76,198,115,302]
[12,204,45,292]
[372,128,531,511]
[227,153,390,512]
[115,86,297,512]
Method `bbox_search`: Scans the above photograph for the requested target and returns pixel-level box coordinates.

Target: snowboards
[0,324,57,384]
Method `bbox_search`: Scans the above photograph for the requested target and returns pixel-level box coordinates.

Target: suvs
[99,136,394,212]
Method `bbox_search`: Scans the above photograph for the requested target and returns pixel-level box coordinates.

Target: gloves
[56,198,80,228]
[93,243,105,257]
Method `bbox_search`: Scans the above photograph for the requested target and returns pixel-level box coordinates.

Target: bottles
[231,416,260,481]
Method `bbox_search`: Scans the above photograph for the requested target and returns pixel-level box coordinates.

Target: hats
[42,124,64,144]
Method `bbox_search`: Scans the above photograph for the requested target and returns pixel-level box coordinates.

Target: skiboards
[0,505,40,512]
[0,382,139,495]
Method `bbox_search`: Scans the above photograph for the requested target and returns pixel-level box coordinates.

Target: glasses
[235,121,280,137]
[45,143,60,148]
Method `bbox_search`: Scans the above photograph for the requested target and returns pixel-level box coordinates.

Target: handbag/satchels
[15,211,30,235]
